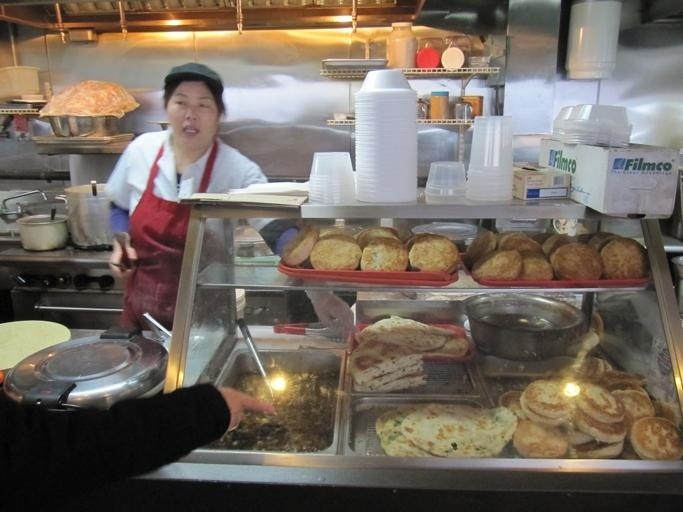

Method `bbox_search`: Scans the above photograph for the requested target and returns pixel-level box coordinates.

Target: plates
[409,221,479,238]
[10,97,49,105]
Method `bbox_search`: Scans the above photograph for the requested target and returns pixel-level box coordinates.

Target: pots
[457,290,587,363]
[2,326,170,414]
[55,183,116,252]
[234,224,274,255]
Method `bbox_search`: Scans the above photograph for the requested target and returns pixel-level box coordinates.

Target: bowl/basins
[549,103,633,147]
[424,158,466,198]
[17,213,69,251]
[1,318,71,383]
[307,150,355,203]
[464,115,514,202]
[354,70,419,204]
[46,116,123,138]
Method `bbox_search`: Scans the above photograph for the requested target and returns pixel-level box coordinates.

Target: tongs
[272,318,346,342]
[108,231,132,271]
[142,311,176,345]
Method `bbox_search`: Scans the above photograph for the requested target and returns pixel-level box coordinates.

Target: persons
[1,383,275,511]
[105,63,354,332]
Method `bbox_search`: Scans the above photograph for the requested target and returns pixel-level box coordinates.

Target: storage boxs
[538,135,680,221]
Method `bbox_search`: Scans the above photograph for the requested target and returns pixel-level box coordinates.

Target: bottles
[386,20,418,72]
[429,89,450,122]
[418,37,445,68]
[442,35,473,69]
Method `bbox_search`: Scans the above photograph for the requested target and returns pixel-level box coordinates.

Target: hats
[164,63,224,94]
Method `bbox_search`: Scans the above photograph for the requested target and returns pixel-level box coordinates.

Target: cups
[453,103,474,119]
[416,98,430,121]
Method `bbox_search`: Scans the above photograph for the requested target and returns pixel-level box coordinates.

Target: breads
[467,226,648,281]
[499,369,682,458]
[284,225,463,273]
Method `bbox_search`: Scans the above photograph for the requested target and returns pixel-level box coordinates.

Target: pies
[349,336,428,395]
[358,315,474,358]
[362,400,517,458]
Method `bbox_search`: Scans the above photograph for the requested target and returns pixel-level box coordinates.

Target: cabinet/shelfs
[319,58,503,130]
[161,185,683,491]
